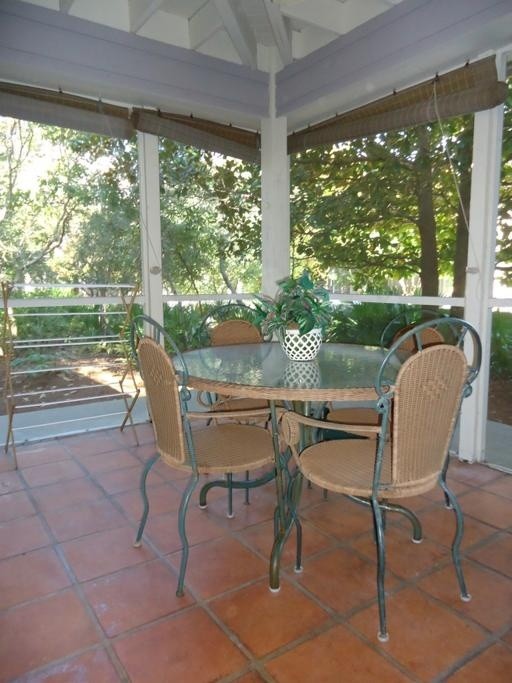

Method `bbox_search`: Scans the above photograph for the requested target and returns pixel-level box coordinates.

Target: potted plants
[249,265,333,361]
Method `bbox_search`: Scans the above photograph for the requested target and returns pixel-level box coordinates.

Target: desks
[167,343,423,596]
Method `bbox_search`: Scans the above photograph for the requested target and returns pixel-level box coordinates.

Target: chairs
[197,303,292,506]
[128,314,303,597]
[325,309,465,444]
[287,317,481,641]
